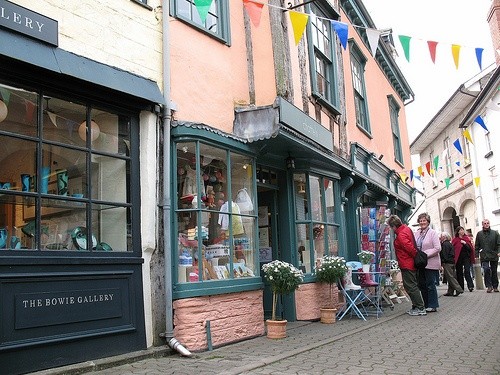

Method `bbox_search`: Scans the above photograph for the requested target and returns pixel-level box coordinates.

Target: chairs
[336,261,411,321]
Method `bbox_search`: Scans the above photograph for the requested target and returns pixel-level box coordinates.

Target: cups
[0,229,7,249]
[20,172,32,191]
[1,181,10,190]
[41,168,50,193]
[56,168,68,195]
[10,236,21,249]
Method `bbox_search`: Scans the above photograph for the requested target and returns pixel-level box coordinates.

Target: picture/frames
[23,174,86,221]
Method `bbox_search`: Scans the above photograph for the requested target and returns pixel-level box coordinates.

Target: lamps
[242,163,249,170]
[182,146,191,154]
[405,177,408,181]
[79,119,100,141]
[284,153,295,170]
[379,155,383,161]
[0,99,9,122]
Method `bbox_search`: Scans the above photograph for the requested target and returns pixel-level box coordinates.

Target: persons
[387,213,500,316]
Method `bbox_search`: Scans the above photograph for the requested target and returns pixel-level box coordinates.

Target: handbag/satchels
[408,227,428,269]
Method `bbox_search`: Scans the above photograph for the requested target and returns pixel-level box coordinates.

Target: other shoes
[454,290,464,297]
[494,289,499,292]
[406,308,427,316]
[487,287,492,293]
[426,307,436,312]
[469,287,473,292]
[444,291,453,296]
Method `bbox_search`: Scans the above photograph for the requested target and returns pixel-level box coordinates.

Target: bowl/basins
[72,194,84,198]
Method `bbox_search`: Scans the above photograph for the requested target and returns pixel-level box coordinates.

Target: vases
[266,320,288,339]
[363,265,370,272]
[319,308,338,324]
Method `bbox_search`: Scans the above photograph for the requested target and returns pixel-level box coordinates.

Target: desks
[350,272,385,319]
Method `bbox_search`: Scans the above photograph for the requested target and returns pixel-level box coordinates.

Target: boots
[358,267,379,287]
[341,267,361,290]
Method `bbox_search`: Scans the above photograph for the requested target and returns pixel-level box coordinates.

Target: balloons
[79,120,100,142]
[0,100,8,123]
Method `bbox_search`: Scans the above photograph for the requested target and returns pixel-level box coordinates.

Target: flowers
[357,250,375,265]
[311,255,349,309]
[262,260,306,321]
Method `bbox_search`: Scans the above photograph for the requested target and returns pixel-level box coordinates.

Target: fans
[43,98,66,115]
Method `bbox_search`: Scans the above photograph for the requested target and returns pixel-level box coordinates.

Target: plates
[71,226,97,250]
[96,243,113,250]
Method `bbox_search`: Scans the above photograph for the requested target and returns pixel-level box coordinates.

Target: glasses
[484,223,489,224]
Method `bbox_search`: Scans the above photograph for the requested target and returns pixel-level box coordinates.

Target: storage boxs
[178,228,249,283]
